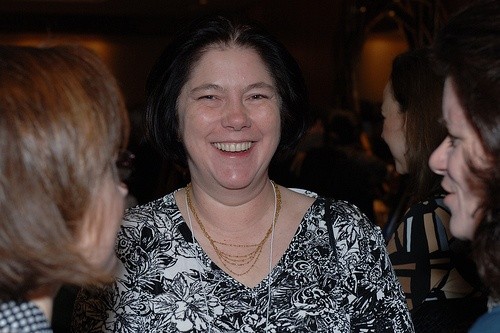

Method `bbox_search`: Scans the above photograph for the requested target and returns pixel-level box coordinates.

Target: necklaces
[184,176,281,333]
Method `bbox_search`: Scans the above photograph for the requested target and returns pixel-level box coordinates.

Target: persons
[379,30,498,333]
[72,26,415,333]
[0,43,136,333]
